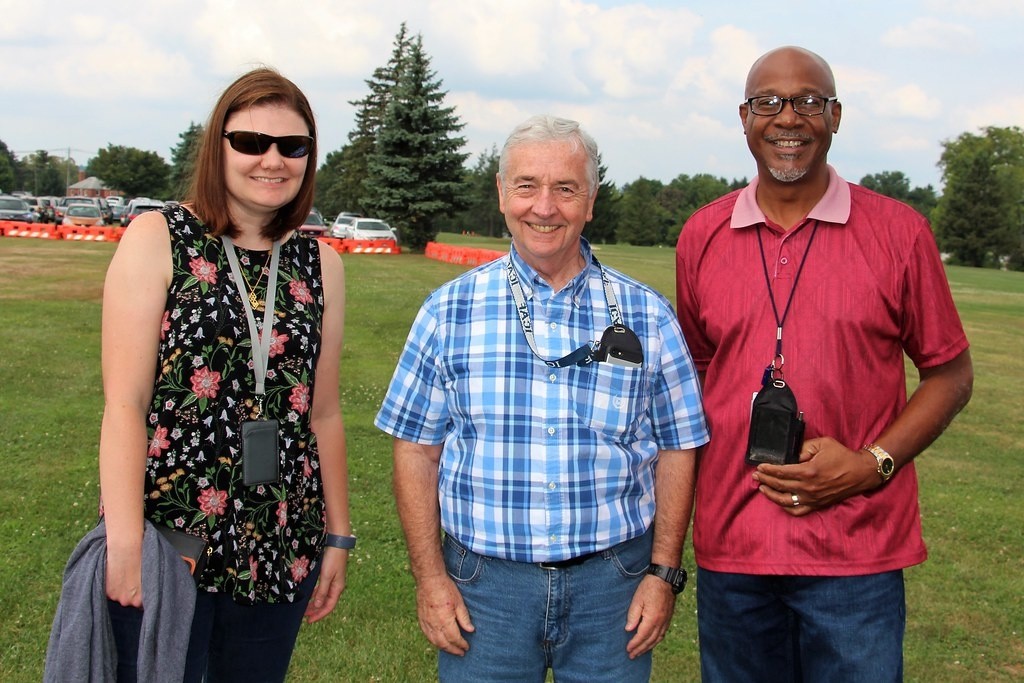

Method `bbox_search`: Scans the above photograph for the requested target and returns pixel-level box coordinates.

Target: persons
[375,117,711,683]
[676,46,974,683]
[98,68,358,683]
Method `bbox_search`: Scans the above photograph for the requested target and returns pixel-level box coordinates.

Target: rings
[659,633,666,637]
[791,492,800,507]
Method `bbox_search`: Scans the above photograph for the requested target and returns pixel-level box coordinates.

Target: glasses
[743,96,838,117]
[221,129,315,157]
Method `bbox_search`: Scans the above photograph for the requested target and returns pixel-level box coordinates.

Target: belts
[538,551,600,570]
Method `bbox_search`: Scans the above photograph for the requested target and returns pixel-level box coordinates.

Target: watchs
[862,444,894,485]
[648,563,688,595]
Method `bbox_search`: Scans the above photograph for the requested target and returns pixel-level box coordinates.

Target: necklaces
[238,245,272,310]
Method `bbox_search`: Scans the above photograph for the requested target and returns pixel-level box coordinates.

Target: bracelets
[327,532,357,549]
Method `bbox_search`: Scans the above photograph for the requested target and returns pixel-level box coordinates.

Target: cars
[339,212,363,220]
[344,217,398,247]
[295,211,331,239]
[0,190,179,230]
[330,215,359,239]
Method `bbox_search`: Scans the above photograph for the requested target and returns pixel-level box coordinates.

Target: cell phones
[605,344,643,369]
